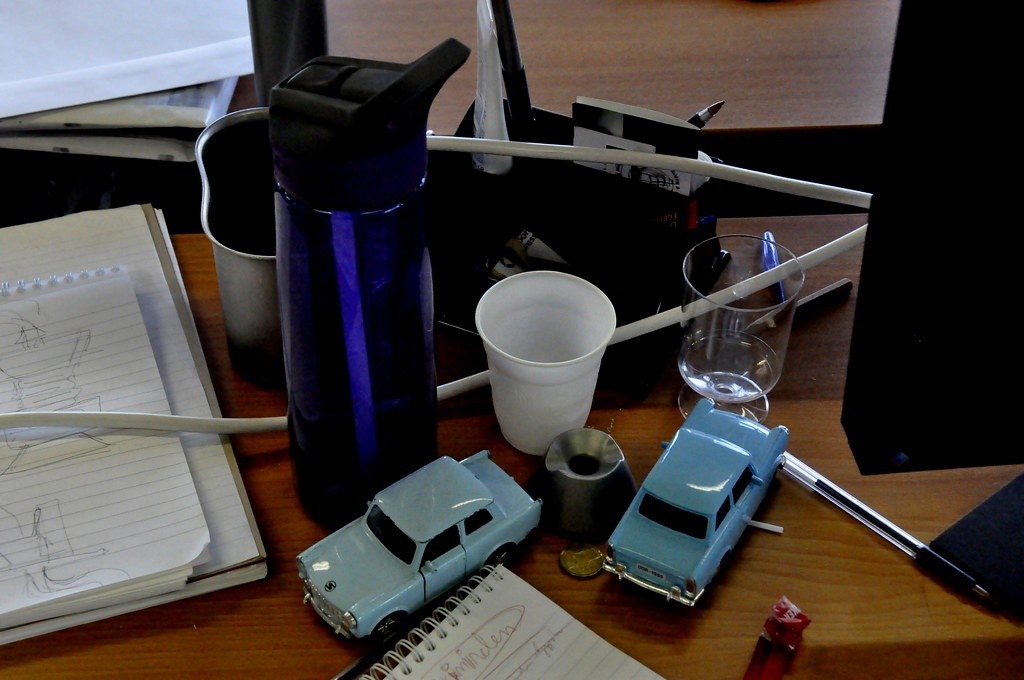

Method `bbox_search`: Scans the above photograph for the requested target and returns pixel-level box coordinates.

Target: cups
[475,270,617,455]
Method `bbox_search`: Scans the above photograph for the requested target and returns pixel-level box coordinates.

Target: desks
[0,212,1024,680]
[322,0,903,141]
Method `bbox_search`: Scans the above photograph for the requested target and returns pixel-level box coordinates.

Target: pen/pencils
[490,0,535,138]
[779,452,990,606]
[764,232,787,306]
[688,100,725,130]
[739,278,852,339]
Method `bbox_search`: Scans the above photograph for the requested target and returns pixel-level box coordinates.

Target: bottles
[266,37,470,534]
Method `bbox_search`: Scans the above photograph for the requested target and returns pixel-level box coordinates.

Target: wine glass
[678,233,805,426]
